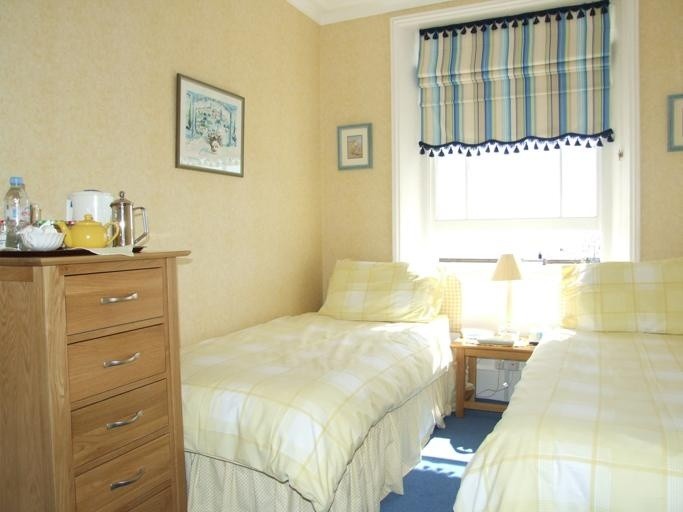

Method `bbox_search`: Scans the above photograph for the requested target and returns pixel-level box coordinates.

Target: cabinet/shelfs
[1,244,191,512]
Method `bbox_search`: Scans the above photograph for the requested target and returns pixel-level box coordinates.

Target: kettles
[65,190,114,248]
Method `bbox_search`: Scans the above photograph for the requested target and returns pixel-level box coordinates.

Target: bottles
[0,176,42,248]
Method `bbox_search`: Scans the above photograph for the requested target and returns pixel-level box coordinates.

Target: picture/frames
[337,121,373,170]
[667,94,683,153]
[176,73,245,177]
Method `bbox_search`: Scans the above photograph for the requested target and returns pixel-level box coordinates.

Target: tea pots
[110,190,148,249]
[57,215,117,248]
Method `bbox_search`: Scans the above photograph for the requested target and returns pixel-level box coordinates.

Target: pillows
[409,273,444,323]
[562,260,683,336]
[318,259,409,321]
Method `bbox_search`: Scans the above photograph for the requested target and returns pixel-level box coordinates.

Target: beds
[180,275,465,512]
[455,309,683,512]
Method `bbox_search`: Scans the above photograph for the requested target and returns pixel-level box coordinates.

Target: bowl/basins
[17,231,64,250]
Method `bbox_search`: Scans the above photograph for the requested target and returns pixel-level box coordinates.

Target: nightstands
[448,331,536,420]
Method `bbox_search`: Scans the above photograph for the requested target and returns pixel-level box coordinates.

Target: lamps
[492,251,521,346]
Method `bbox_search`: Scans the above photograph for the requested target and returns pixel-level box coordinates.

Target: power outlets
[508,359,519,371]
[495,359,504,370]
